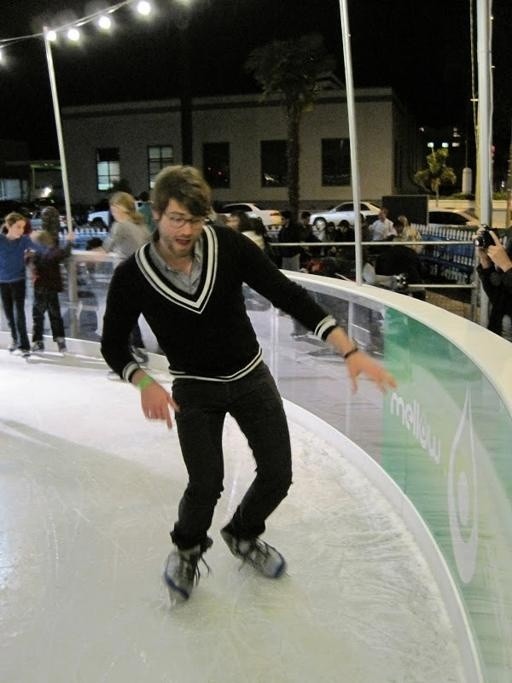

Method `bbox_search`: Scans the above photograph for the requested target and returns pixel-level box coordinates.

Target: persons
[101,166,399,609]
[470,194,511,341]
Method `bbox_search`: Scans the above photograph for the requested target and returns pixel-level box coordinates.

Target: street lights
[427,141,440,207]
[452,128,470,169]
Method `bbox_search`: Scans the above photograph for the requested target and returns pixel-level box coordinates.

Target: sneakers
[217,525,289,581]
[161,532,215,605]
[129,345,151,363]
[7,334,68,357]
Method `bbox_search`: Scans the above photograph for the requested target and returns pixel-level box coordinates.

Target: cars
[220,202,286,225]
[308,199,382,230]
[1,195,75,234]
[86,199,150,227]
[409,206,479,230]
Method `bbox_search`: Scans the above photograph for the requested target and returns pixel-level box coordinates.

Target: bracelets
[343,347,358,358]
[136,374,154,392]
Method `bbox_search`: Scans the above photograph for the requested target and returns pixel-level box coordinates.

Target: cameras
[476,228,498,248]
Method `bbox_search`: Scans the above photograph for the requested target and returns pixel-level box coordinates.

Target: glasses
[160,210,212,230]
